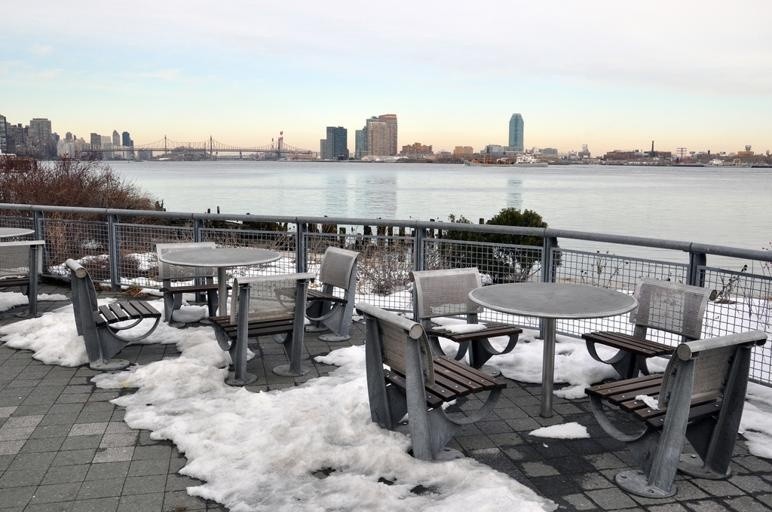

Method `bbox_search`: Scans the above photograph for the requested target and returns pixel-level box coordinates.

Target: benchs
[0,240,47,319]
[584,329,767,498]
[64,242,234,372]
[353,268,523,462]
[209,245,362,386]
[582,278,714,380]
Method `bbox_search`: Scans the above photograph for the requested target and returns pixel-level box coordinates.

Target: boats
[462,147,548,168]
[625,157,671,166]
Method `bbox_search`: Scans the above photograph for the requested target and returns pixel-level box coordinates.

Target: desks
[467,281,638,419]
[0,227,36,242]
